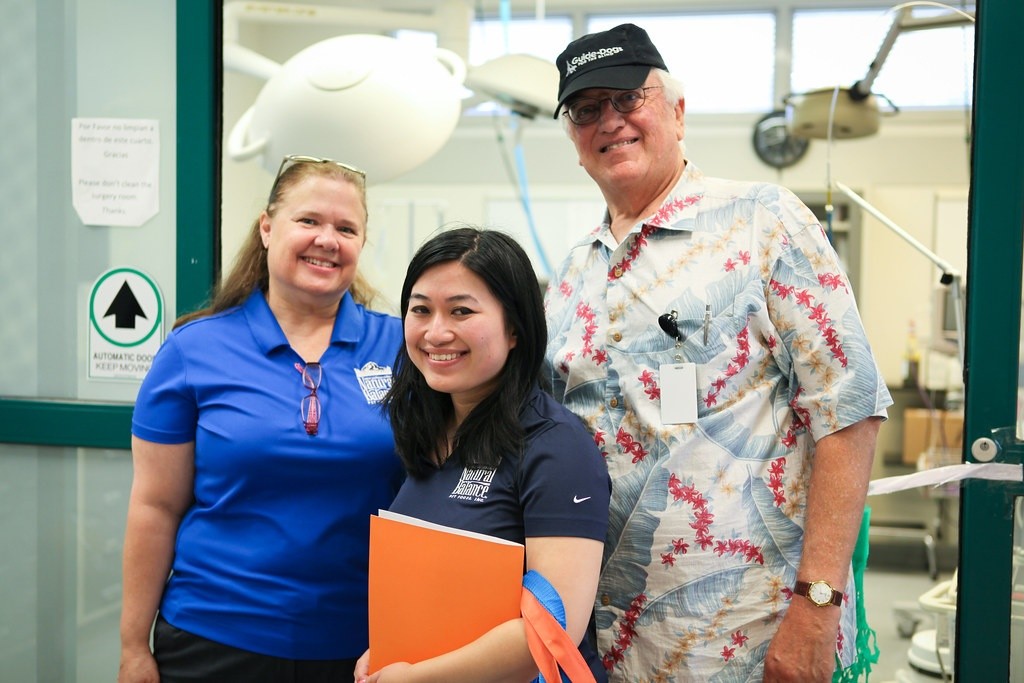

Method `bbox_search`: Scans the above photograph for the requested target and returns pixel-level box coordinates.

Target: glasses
[267,154,366,213]
[294,362,322,435]
[562,84,664,125]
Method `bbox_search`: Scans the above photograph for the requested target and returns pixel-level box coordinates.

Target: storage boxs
[902,406,964,468]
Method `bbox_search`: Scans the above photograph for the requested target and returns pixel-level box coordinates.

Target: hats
[553,23,670,120]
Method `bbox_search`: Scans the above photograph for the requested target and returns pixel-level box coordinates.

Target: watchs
[792,579,843,609]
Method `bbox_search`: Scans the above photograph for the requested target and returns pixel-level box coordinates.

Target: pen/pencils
[703,305,712,346]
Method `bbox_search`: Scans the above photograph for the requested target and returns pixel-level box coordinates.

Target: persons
[120,162,403,683]
[535,23,894,683]
[354,228,613,682]
